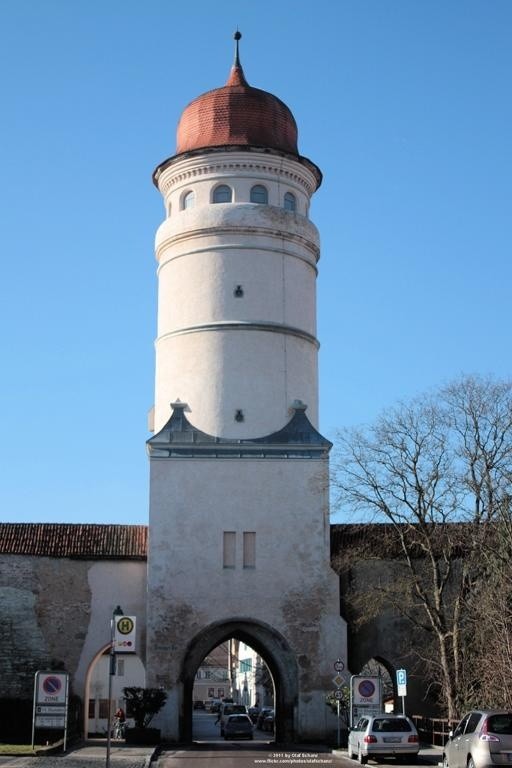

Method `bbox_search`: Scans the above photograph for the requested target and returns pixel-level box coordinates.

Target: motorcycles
[113,716,129,740]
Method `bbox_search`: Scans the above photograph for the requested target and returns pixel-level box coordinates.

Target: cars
[193,697,274,741]
[440,708,512,767]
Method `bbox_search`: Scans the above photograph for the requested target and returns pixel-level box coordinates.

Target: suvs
[346,712,421,764]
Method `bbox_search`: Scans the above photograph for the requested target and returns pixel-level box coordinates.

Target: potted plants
[122,685,168,746]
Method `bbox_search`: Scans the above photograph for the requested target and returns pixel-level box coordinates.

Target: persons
[111,705,127,738]
[254,699,259,707]
[214,703,225,727]
